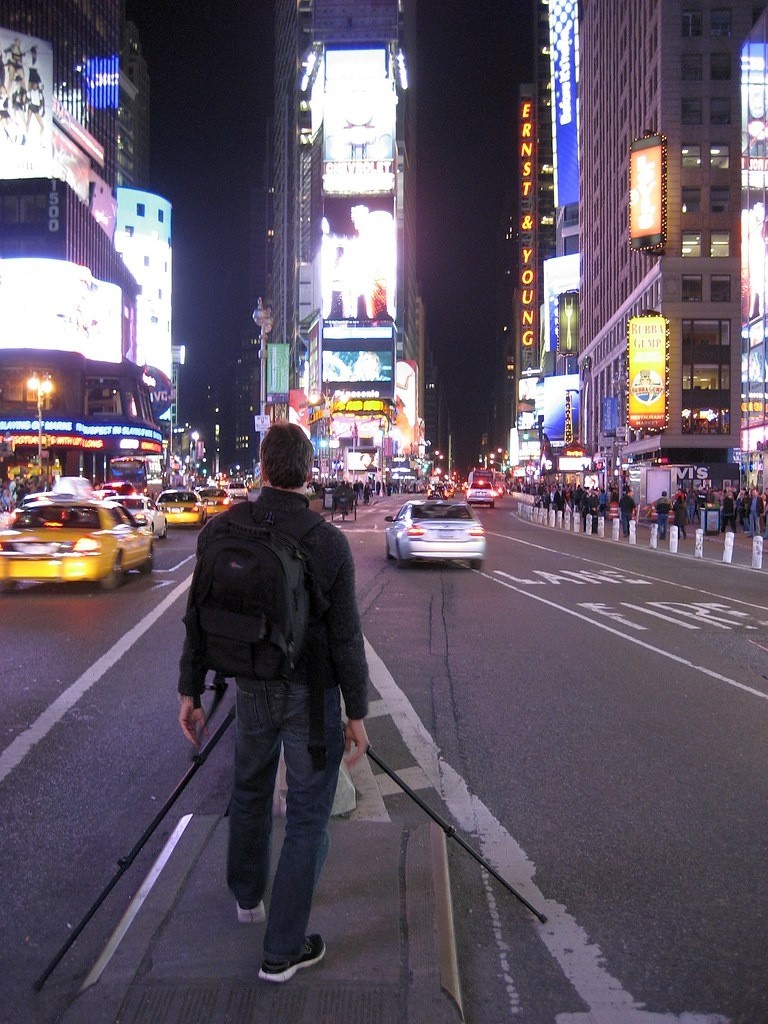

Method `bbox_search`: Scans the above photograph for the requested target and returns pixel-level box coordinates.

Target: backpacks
[195,501,325,681]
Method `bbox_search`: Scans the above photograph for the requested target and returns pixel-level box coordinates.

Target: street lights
[489,448,505,472]
[308,378,350,487]
[522,414,545,449]
[26,370,55,486]
[430,450,445,475]
[252,297,274,491]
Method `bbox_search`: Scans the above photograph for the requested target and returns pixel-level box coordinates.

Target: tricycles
[329,491,357,523]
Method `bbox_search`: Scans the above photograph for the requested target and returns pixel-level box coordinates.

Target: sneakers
[258,934,325,982]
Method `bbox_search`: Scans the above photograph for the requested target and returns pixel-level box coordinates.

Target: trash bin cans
[699,502,720,535]
[324,488,334,508]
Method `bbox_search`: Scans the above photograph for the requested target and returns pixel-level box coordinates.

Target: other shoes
[235,896,266,924]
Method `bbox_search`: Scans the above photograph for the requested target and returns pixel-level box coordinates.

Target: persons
[310,477,431,506]
[180,424,369,983]
[506,478,614,533]
[672,484,768,539]
[0,38,45,147]
[655,492,672,540]
[0,476,36,513]
[619,490,636,537]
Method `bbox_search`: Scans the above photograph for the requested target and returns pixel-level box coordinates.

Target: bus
[110,456,164,500]
[466,469,495,488]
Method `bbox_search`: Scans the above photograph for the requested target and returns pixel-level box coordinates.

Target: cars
[383,500,486,570]
[19,478,168,541]
[196,487,234,517]
[0,501,156,590]
[228,483,249,501]
[465,480,496,508]
[427,483,456,499]
[155,489,210,526]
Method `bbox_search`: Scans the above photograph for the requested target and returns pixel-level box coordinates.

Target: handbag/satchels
[565,504,572,512]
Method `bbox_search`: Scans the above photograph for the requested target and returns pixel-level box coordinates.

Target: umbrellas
[6,465,30,475]
